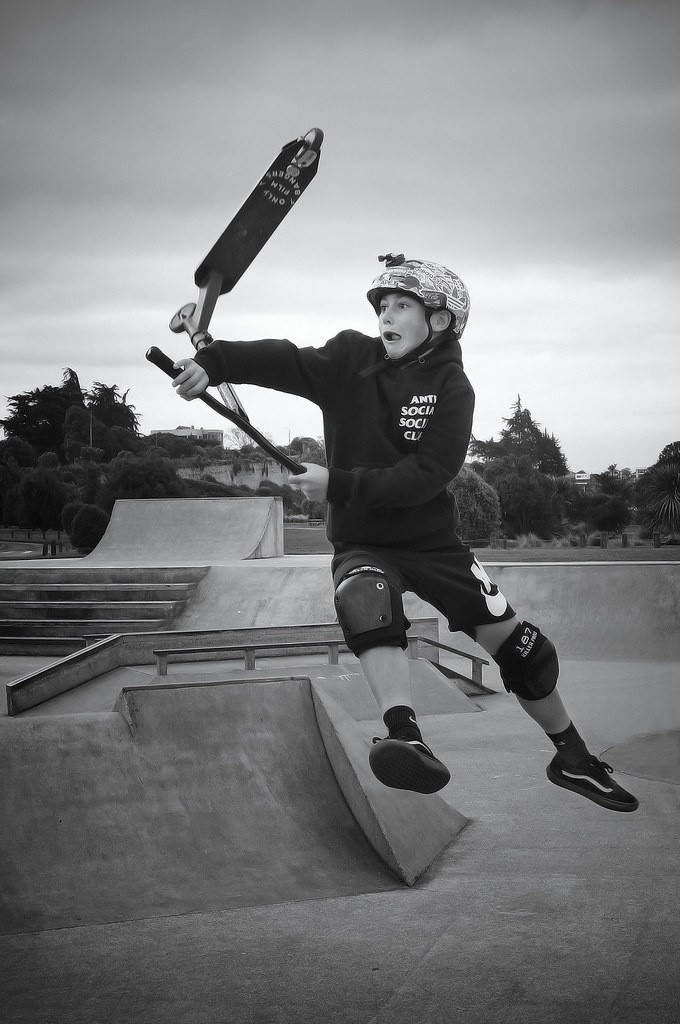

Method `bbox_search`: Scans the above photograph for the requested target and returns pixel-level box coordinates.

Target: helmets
[367,253,470,339]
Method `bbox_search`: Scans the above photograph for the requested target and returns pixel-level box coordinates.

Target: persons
[169,252,641,814]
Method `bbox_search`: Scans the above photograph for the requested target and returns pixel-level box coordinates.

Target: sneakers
[369,736,451,794]
[546,752,639,812]
